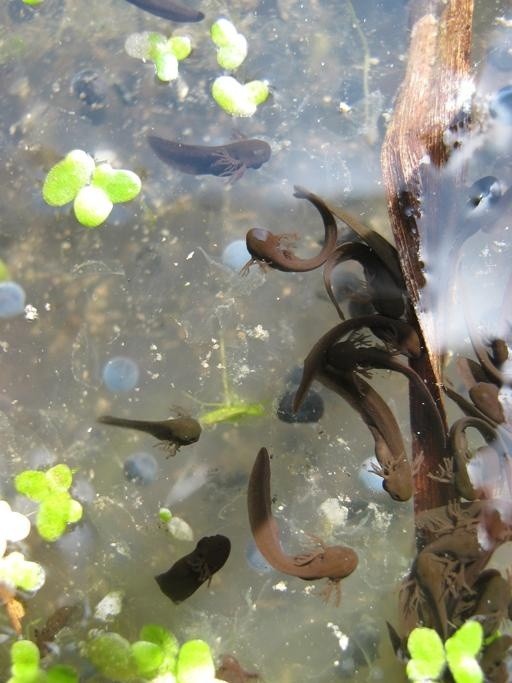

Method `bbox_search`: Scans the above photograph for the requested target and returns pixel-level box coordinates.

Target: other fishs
[214,176,512,683]
